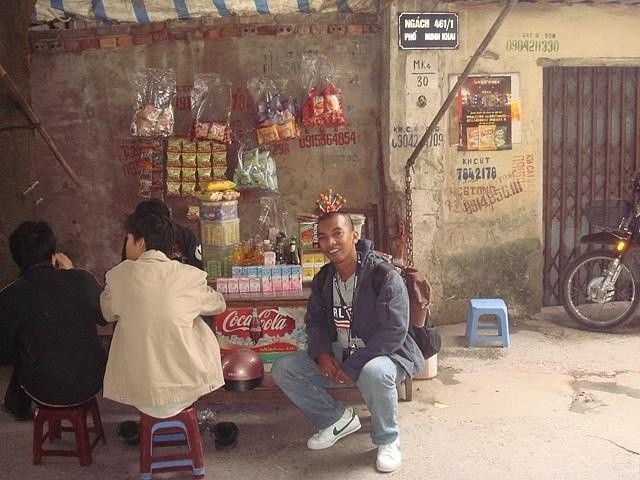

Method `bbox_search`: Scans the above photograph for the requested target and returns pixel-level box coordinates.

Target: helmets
[221,349,264,393]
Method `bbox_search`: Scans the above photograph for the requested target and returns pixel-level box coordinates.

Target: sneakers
[307,406,362,450]
[376,436,401,472]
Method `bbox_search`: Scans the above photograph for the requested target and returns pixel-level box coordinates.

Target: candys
[329,206,334,212]
[335,196,342,208]
[327,188,332,203]
[324,201,330,213]
[334,201,339,209]
[332,193,339,205]
[318,193,324,207]
[324,195,328,209]
[316,199,325,212]
[336,199,346,212]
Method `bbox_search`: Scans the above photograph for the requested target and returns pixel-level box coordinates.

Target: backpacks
[316,258,441,360]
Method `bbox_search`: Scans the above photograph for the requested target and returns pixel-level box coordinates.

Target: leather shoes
[0,405,32,421]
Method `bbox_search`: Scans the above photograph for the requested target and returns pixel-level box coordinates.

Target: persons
[271,212,425,472]
[122,199,203,271]
[100,211,226,418]
[0,220,110,420]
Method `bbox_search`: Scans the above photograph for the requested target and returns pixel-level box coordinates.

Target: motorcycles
[561,199,638,329]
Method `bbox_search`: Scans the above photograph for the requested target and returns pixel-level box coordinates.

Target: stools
[463,298,509,348]
[139,405,205,480]
[32,396,106,467]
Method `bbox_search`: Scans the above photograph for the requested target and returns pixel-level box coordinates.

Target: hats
[401,266,432,328]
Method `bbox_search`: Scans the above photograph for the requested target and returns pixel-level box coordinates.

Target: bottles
[276,233,281,243]
[259,241,264,252]
[292,265,302,289]
[272,265,282,291]
[233,244,238,265]
[255,244,262,265]
[265,251,276,267]
[248,266,256,277]
[246,238,254,256]
[239,277,249,293]
[258,266,262,278]
[217,277,227,294]
[239,242,243,264]
[264,239,270,250]
[263,267,273,291]
[277,243,286,263]
[227,277,238,292]
[291,236,298,254]
[249,307,263,345]
[267,244,272,252]
[232,265,243,276]
[250,277,261,292]
[282,265,292,291]
[289,242,300,263]
[243,240,247,265]
[243,266,247,278]
